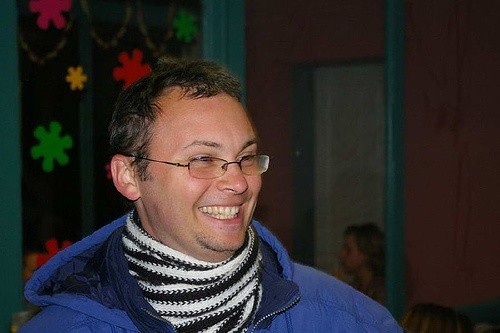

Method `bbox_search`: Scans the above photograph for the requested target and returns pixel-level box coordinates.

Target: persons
[339,223,390,306]
[16,57,404,333]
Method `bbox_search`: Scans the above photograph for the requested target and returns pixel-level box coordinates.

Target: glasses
[128,151,272,179]
